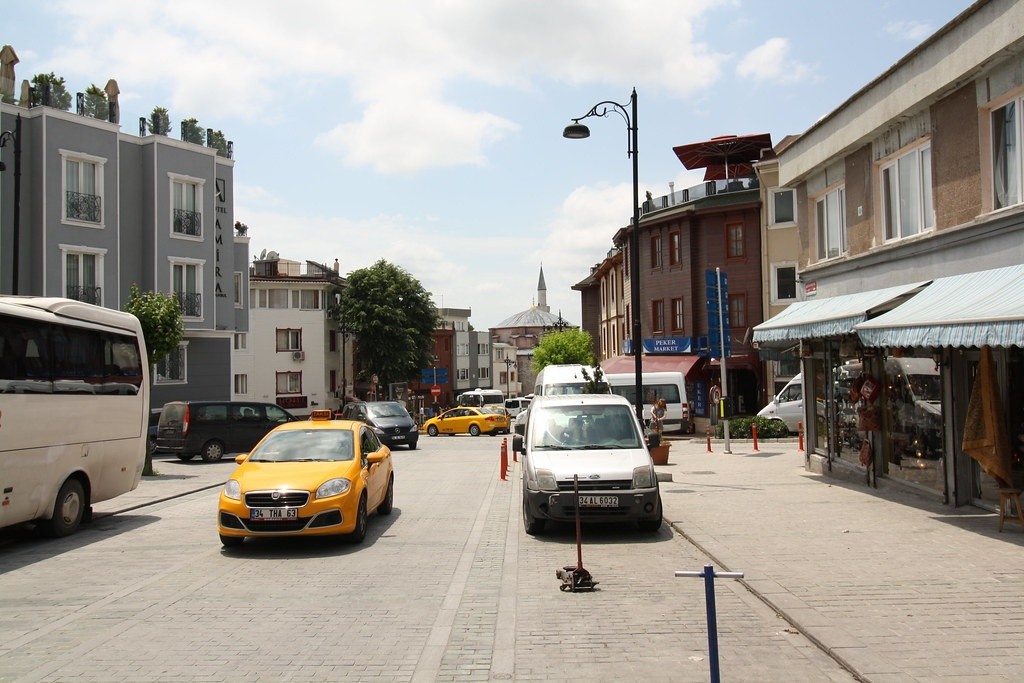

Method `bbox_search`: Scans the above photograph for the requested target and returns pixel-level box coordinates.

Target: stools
[999,489,1024,532]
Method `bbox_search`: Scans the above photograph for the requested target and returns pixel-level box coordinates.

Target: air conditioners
[293,351,305,360]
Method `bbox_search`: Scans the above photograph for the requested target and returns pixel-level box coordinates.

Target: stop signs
[431,385,441,396]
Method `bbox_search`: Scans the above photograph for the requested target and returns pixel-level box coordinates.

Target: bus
[457,388,504,406]
[0,294,149,540]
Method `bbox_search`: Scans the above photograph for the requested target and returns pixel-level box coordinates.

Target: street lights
[504,353,518,399]
[563,86,646,436]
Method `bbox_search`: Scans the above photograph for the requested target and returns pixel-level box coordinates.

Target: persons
[237,409,256,422]
[649,398,667,429]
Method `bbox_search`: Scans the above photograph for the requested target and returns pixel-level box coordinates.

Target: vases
[648,444,671,466]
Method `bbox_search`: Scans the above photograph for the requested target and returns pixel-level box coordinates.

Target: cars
[422,405,506,437]
[216,409,394,547]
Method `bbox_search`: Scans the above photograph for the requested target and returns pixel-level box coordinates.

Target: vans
[505,399,532,419]
[158,401,300,462]
[345,401,419,451]
[512,394,664,536]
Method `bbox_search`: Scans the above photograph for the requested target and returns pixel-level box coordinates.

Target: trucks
[833,356,943,457]
[603,372,690,435]
[534,364,612,395]
[757,371,805,434]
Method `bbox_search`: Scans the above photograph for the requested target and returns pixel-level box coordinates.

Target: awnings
[852,263,1024,349]
[751,280,933,343]
